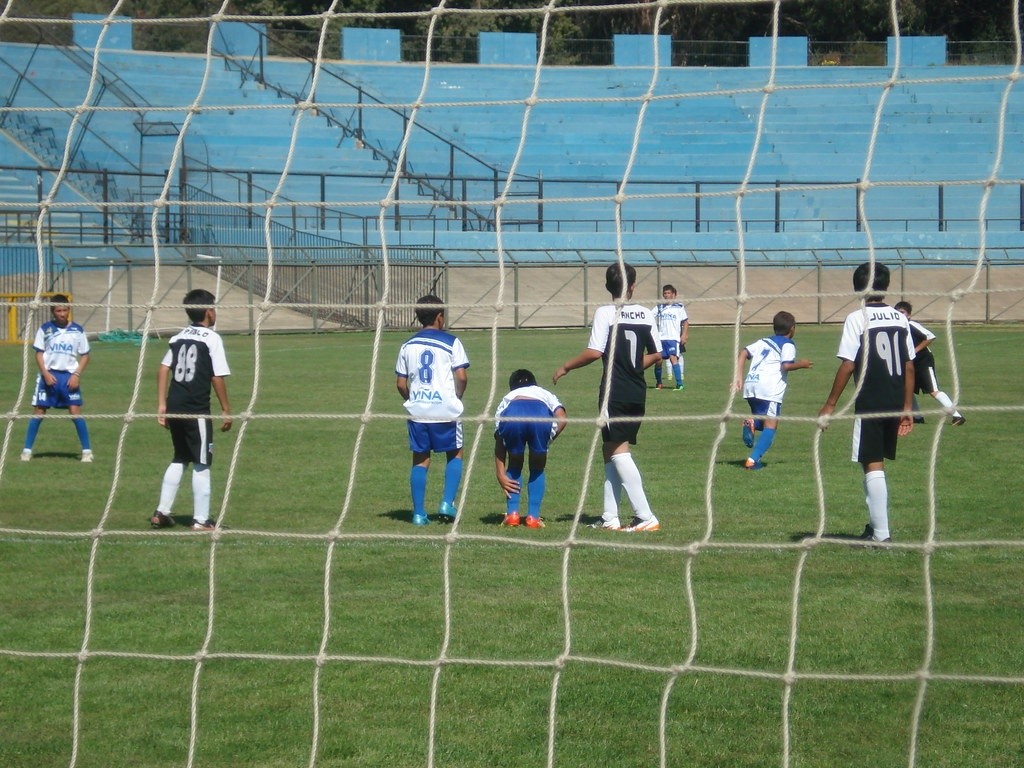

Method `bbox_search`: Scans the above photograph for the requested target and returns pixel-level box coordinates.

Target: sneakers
[525,516,546,528]
[742,418,756,448]
[621,517,661,531]
[588,519,621,530]
[746,459,767,469]
[501,512,521,527]
[413,514,431,525]
[151,510,175,529]
[438,502,457,520]
[192,517,229,531]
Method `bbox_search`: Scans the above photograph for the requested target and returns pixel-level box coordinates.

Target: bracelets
[74,372,81,377]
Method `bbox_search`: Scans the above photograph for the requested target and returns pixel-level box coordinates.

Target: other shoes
[850,524,893,549]
[80,449,95,463]
[913,417,925,424]
[951,416,966,427]
[19,449,32,461]
[655,384,663,390]
[673,385,684,391]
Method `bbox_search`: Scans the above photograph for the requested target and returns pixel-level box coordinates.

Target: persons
[730,311,814,469]
[151,289,233,531]
[395,295,471,525]
[651,285,689,391]
[20,294,94,463]
[553,262,663,533]
[817,261,916,550]
[494,369,568,528]
[894,301,966,427]
